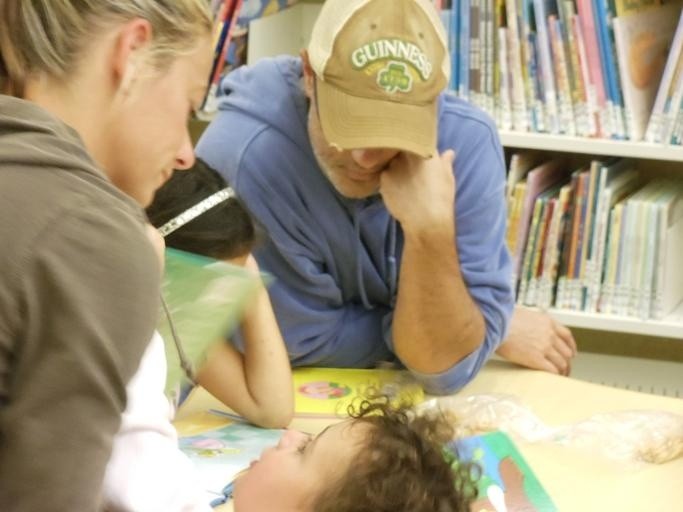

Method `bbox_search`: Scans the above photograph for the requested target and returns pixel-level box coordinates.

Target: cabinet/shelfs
[437,1,682,340]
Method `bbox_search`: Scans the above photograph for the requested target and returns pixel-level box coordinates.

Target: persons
[195,1,576,395]
[0,0,220,512]
[146,159,295,430]
[233,394,482,512]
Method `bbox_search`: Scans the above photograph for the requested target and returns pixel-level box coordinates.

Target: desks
[174,358,682,512]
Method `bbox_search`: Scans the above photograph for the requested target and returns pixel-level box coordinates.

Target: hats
[307,0,452,159]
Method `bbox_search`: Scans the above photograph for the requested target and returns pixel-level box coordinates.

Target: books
[445,429,557,512]
[447,1,683,144]
[292,366,425,419]
[506,153,682,318]
[172,409,315,507]
[194,1,325,114]
